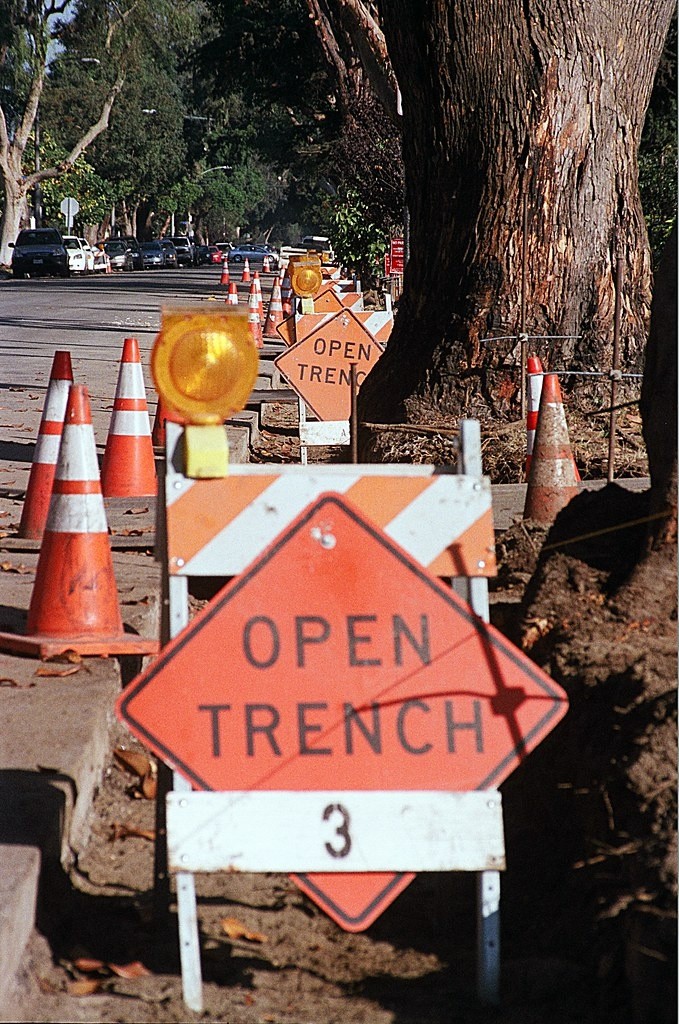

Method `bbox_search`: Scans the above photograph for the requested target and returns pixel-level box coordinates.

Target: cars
[138,234,236,272]
[256,243,298,270]
[228,244,280,264]
[93,240,132,271]
[61,235,91,276]
[64,237,107,275]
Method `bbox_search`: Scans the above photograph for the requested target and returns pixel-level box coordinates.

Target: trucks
[301,236,339,265]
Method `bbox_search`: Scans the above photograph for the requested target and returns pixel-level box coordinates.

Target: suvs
[8,227,71,280]
[104,234,147,271]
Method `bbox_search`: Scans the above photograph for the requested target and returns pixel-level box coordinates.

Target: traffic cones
[524,355,544,485]
[263,256,295,319]
[520,373,584,534]
[262,276,284,339]
[0,378,160,663]
[99,335,159,501]
[225,282,239,305]
[240,257,252,283]
[217,256,232,287]
[251,270,266,322]
[245,283,265,349]
[0,349,72,548]
